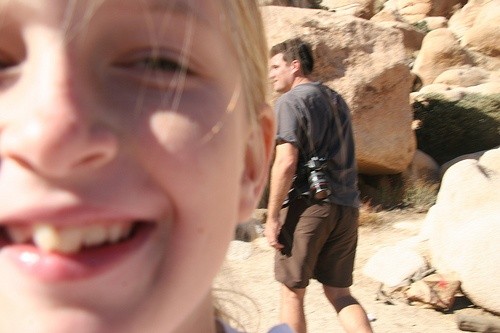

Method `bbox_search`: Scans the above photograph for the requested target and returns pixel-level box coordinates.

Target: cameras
[303,157,333,202]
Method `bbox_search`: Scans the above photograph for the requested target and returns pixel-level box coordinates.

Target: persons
[263,37,374,333]
[1,0,295,333]
